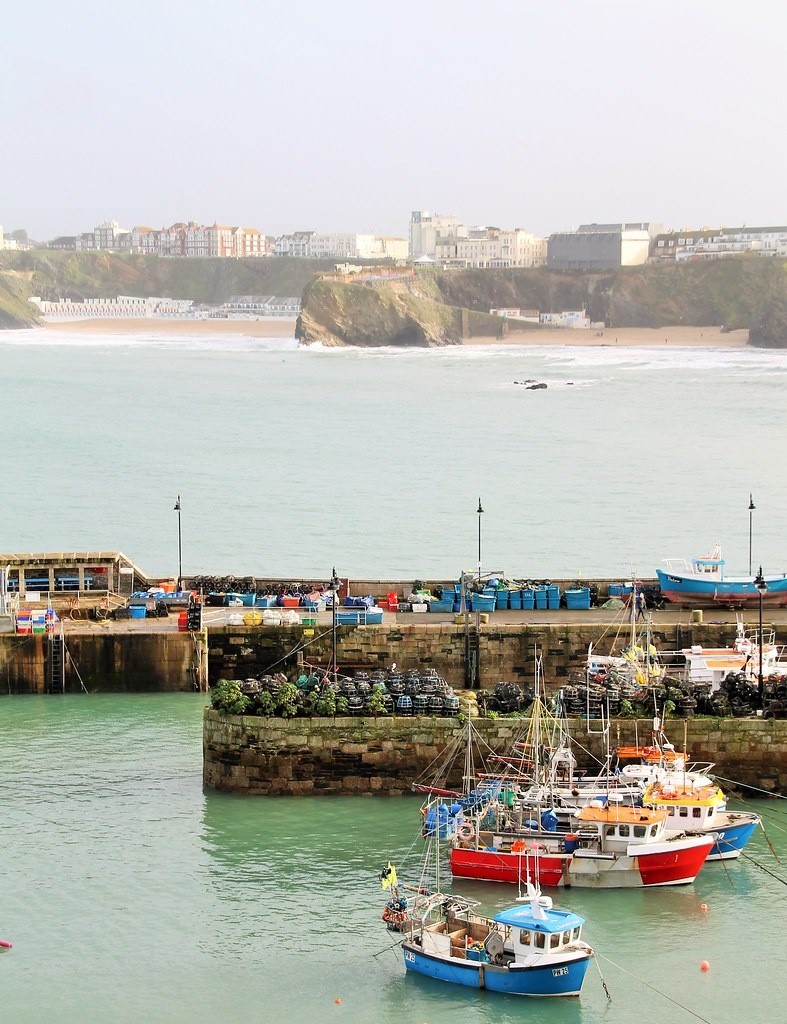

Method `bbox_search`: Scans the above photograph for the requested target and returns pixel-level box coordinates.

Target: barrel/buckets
[526,810,558,832]
[427,787,514,837]
[565,833,579,853]
[693,610,703,622]
[483,846,497,851]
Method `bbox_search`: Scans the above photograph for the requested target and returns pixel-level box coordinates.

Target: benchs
[7,577,93,592]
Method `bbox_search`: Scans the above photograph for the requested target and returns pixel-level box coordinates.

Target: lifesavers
[457,823,475,840]
[564,834,579,841]
[664,793,680,800]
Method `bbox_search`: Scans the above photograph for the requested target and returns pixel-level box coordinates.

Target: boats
[411,577,760,890]
[587,582,663,681]
[656,538,787,610]
[682,618,787,686]
[377,669,593,998]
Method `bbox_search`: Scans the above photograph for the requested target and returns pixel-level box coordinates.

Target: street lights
[747,493,756,577]
[754,566,768,695]
[476,498,484,582]
[174,495,182,593]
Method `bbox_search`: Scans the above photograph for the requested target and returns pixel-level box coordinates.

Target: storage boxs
[302,618,317,624]
[17,609,56,633]
[334,611,384,625]
[607,580,641,605]
[377,579,599,613]
[113,582,201,631]
[424,780,514,837]
[208,591,327,613]
[542,814,558,831]
[510,839,526,852]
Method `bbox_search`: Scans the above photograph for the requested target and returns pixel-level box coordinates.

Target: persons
[590,586,598,607]
[628,588,647,621]
[521,931,530,945]
